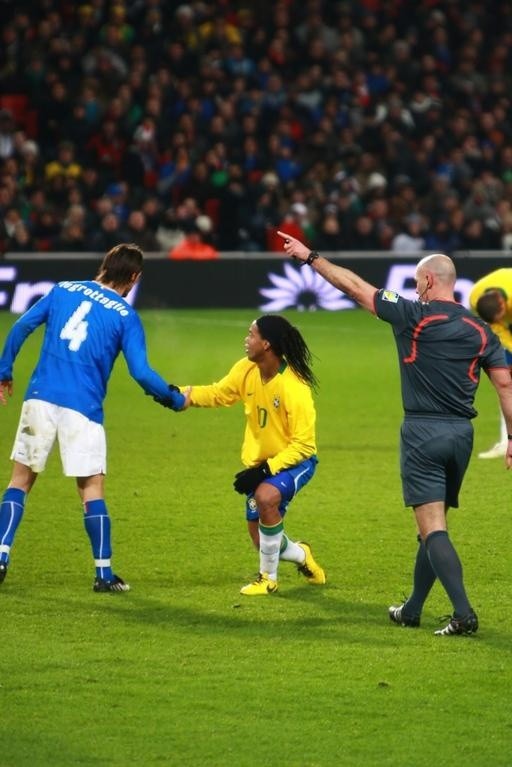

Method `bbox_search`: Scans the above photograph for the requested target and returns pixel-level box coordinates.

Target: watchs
[307,251,318,265]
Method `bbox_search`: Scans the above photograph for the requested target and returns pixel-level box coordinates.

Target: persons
[0,242,193,594]
[276,230,512,636]
[469,267,512,459]
[153,314,326,595]
[1,1,512,254]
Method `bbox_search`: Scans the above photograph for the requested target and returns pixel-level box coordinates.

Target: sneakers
[434,606,481,637]
[239,572,279,597]
[294,542,326,583]
[92,572,132,593]
[388,604,421,629]
[478,443,509,459]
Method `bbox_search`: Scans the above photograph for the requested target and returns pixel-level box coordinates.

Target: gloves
[232,459,273,495]
[145,385,180,406]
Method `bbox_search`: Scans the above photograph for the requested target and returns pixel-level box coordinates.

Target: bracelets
[507,434,512,440]
